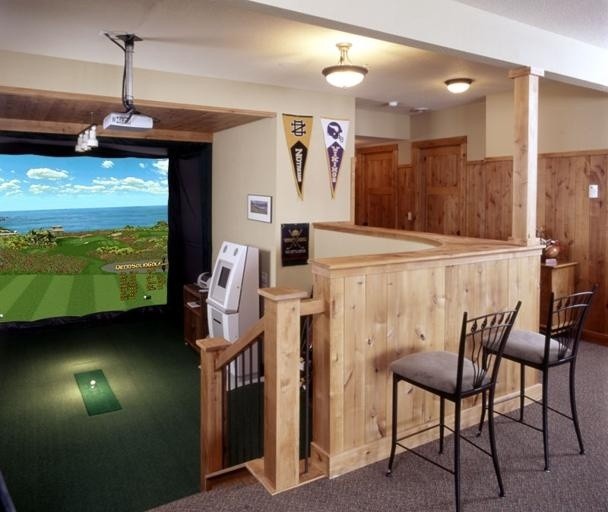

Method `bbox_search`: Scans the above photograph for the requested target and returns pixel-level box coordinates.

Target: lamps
[443,76,474,95]
[74,112,98,153]
[320,41,369,89]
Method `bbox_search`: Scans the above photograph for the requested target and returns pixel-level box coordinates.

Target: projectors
[102,112,153,133]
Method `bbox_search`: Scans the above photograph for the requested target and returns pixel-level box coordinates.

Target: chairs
[474,282,599,473]
[384,300,523,512]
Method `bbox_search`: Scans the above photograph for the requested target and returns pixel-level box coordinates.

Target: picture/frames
[245,193,273,224]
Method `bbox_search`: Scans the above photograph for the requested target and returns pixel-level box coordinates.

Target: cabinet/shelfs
[181,282,208,354]
[541,260,578,338]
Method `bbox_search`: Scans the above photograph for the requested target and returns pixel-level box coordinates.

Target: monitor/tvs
[218,266,231,288]
